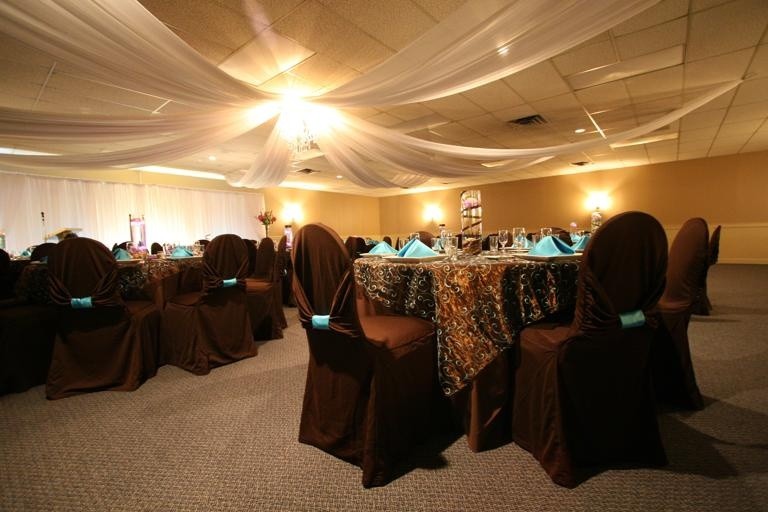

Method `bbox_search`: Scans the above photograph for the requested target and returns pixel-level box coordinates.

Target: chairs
[1,234,289,401]
[289,207,723,488]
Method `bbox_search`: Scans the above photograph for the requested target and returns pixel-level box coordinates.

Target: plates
[359,253,394,258]
[384,256,448,263]
[112,260,140,265]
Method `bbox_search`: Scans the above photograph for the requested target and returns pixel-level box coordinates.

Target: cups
[162,237,201,256]
[396,226,587,263]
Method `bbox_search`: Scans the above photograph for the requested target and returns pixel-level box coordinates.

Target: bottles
[439,223,446,237]
[284,224,293,249]
